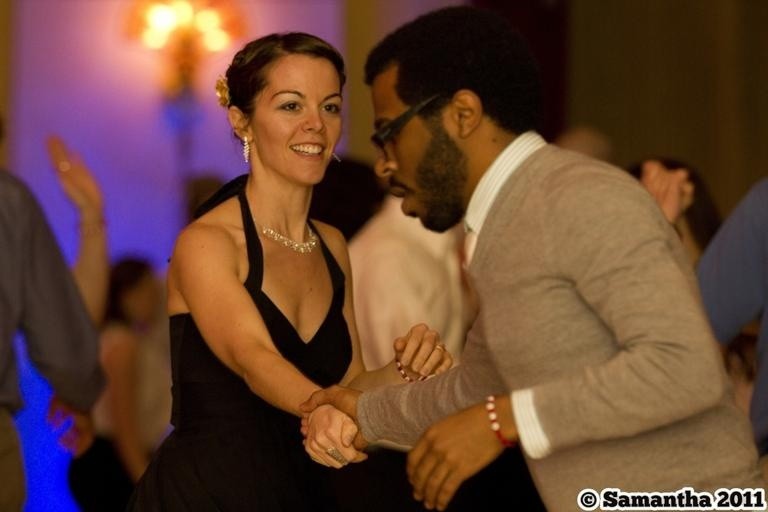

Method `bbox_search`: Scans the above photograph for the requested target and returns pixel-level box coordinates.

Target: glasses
[366,88,459,149]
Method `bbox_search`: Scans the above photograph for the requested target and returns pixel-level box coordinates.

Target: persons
[121,31,456,510]
[1,113,768,512]
[297,0,767,511]
